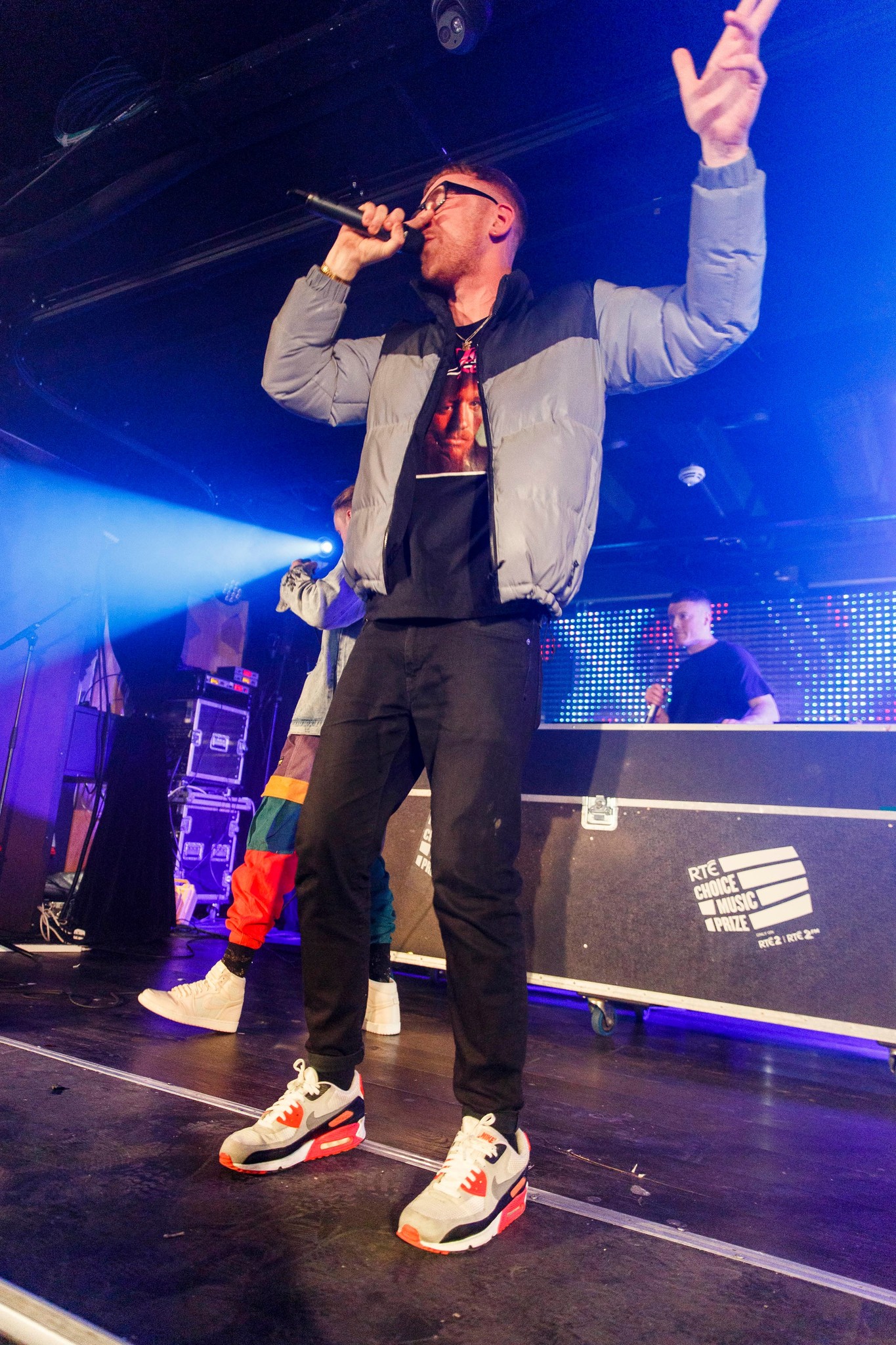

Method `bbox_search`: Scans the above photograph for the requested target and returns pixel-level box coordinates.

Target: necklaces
[447,309,497,352]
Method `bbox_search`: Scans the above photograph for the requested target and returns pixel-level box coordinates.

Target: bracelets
[319,262,351,289]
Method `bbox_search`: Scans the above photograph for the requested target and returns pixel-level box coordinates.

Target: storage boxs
[166,793,249,908]
[156,697,250,786]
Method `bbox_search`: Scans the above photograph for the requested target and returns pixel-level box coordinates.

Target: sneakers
[218,1057,367,1176]
[396,1114,533,1254]
[138,960,246,1032]
[360,977,401,1036]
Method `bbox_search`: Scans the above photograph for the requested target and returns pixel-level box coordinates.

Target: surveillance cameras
[431,0,486,56]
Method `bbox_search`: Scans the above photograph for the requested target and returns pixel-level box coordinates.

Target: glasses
[410,180,498,220]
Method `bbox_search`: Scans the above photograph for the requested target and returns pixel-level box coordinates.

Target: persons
[137,481,405,1036]
[213,0,785,1259]
[644,584,782,727]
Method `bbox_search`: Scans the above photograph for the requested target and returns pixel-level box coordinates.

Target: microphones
[284,187,424,256]
[645,704,659,724]
